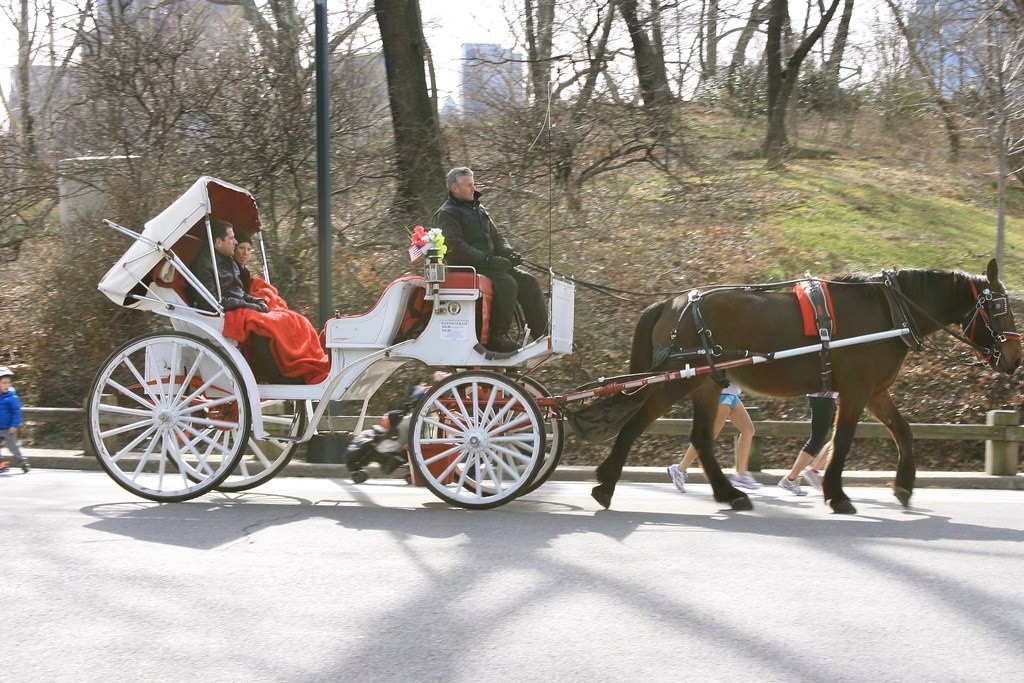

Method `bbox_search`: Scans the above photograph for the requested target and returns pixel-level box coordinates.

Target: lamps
[425,248,445,283]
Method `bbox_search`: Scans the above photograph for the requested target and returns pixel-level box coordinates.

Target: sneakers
[730,474,760,489]
[667,464,688,493]
[778,476,807,496]
[800,465,822,491]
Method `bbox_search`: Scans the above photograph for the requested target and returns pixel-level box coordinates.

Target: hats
[234,231,252,246]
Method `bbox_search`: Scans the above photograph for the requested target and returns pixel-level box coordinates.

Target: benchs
[437,265,493,296]
[322,275,426,347]
[151,233,221,318]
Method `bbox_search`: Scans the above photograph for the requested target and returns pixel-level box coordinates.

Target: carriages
[87,174,1022,514]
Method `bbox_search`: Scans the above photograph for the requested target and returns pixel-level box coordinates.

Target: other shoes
[22,463,31,472]
[269,375,303,384]
[489,334,521,352]
[531,328,552,340]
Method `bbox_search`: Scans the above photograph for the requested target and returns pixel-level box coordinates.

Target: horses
[591,259,1023,515]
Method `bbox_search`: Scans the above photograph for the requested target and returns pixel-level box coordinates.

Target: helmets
[0,366,14,376]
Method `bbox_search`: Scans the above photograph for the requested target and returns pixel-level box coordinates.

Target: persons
[187,218,305,384]
[778,389,839,497]
[346,409,404,462]
[0,366,30,474]
[431,166,552,353]
[667,381,764,495]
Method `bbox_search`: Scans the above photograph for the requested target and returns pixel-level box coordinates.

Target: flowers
[404,225,448,265]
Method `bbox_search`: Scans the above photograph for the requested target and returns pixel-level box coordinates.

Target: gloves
[244,303,261,311]
[509,252,522,266]
[257,300,268,311]
[486,255,511,273]
